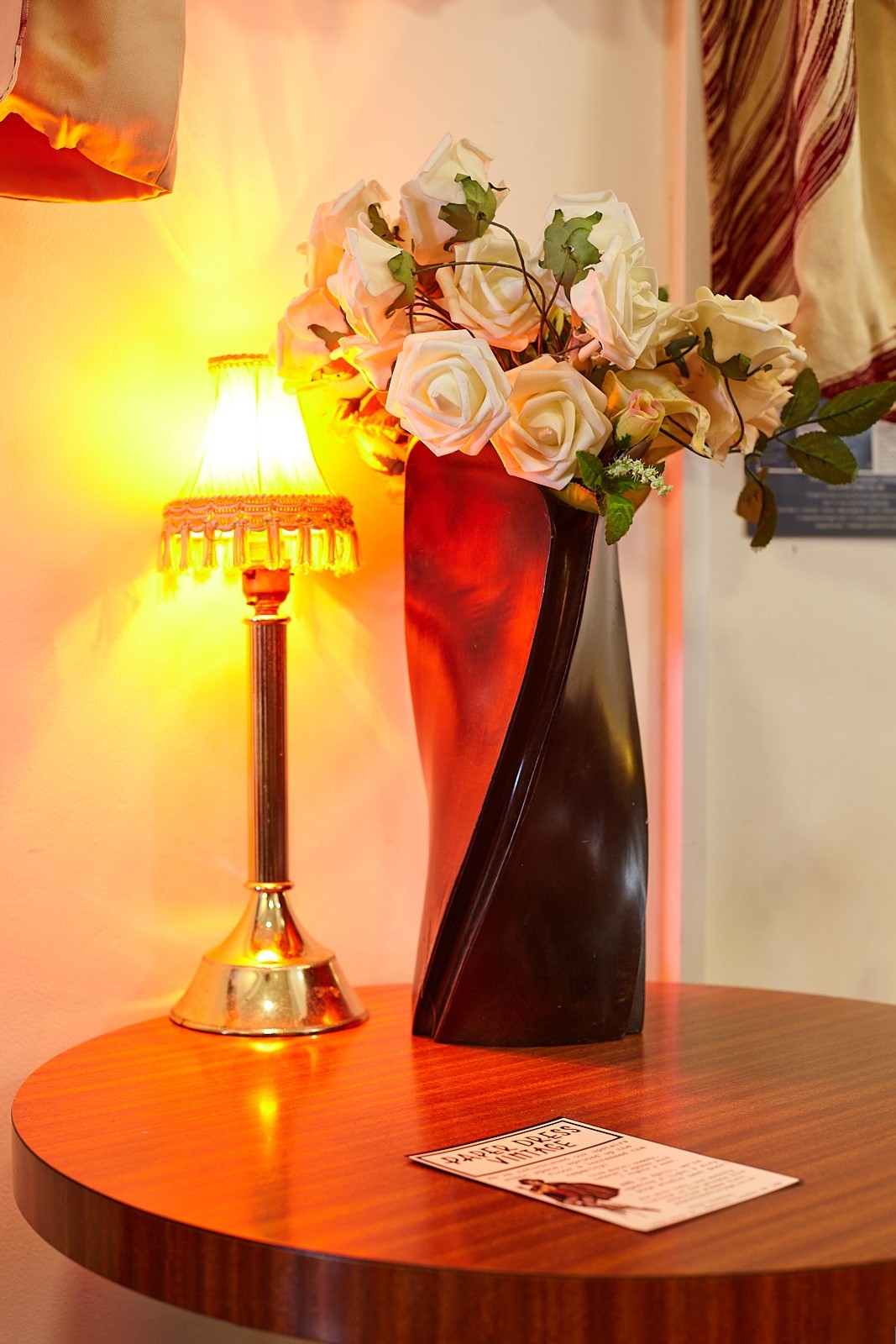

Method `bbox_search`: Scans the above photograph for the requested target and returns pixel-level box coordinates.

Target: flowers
[283,134,896,546]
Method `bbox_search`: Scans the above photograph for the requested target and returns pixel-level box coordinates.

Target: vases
[402,437,652,1049]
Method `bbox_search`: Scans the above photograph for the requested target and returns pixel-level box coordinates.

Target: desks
[11,981,896,1344]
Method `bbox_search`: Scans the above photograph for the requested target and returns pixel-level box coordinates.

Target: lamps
[156,348,367,1038]
